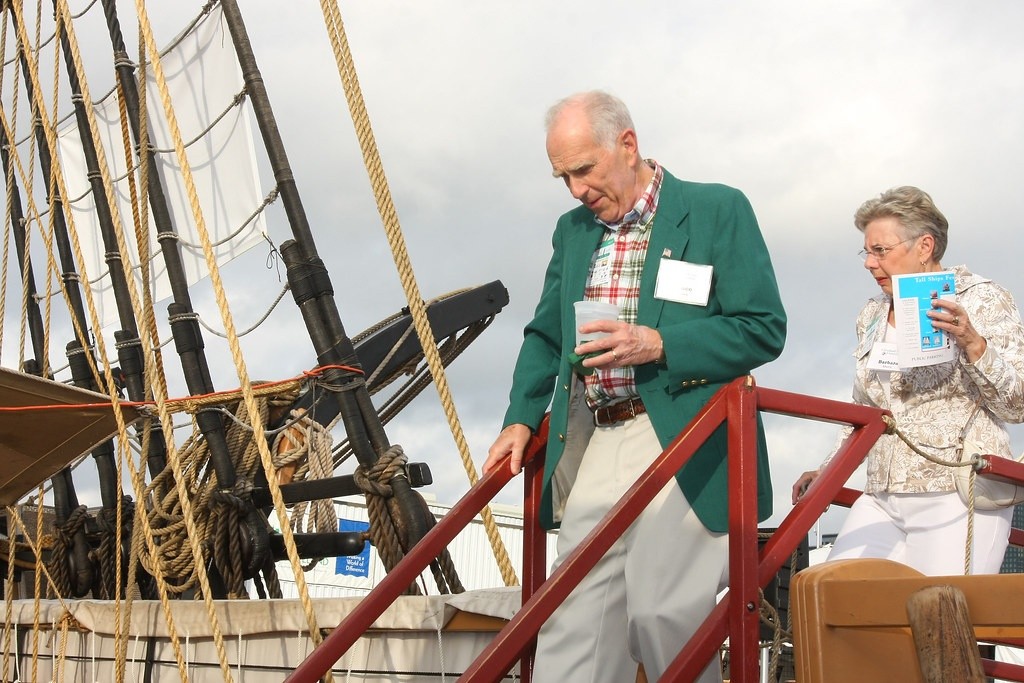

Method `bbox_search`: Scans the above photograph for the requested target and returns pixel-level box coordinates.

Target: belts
[589,398,648,428]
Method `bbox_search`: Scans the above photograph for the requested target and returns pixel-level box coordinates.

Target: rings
[952,316,960,326]
[611,350,618,361]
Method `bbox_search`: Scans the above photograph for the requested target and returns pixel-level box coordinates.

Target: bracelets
[654,336,667,367]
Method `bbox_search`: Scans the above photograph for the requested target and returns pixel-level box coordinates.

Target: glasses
[857,235,921,260]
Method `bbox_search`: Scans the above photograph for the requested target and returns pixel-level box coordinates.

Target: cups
[572,301,622,348]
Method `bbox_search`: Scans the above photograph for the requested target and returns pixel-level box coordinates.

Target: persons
[793,183,1024,580]
[480,90,789,683]
